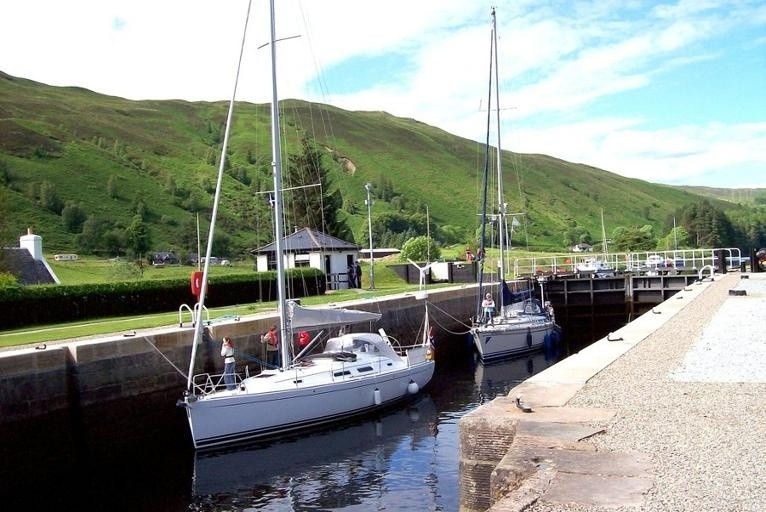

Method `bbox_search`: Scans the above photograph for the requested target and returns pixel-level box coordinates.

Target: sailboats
[469,5,556,363]
[176,0,436,454]
[576,202,615,278]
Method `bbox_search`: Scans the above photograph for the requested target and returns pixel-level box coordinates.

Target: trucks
[645,255,682,275]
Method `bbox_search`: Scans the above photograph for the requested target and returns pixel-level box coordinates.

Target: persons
[351,262,359,288]
[348,265,357,288]
[260,325,281,370]
[354,261,363,288]
[297,326,310,362]
[466,246,474,260]
[481,292,496,328]
[220,336,236,390]
[476,244,484,260]
